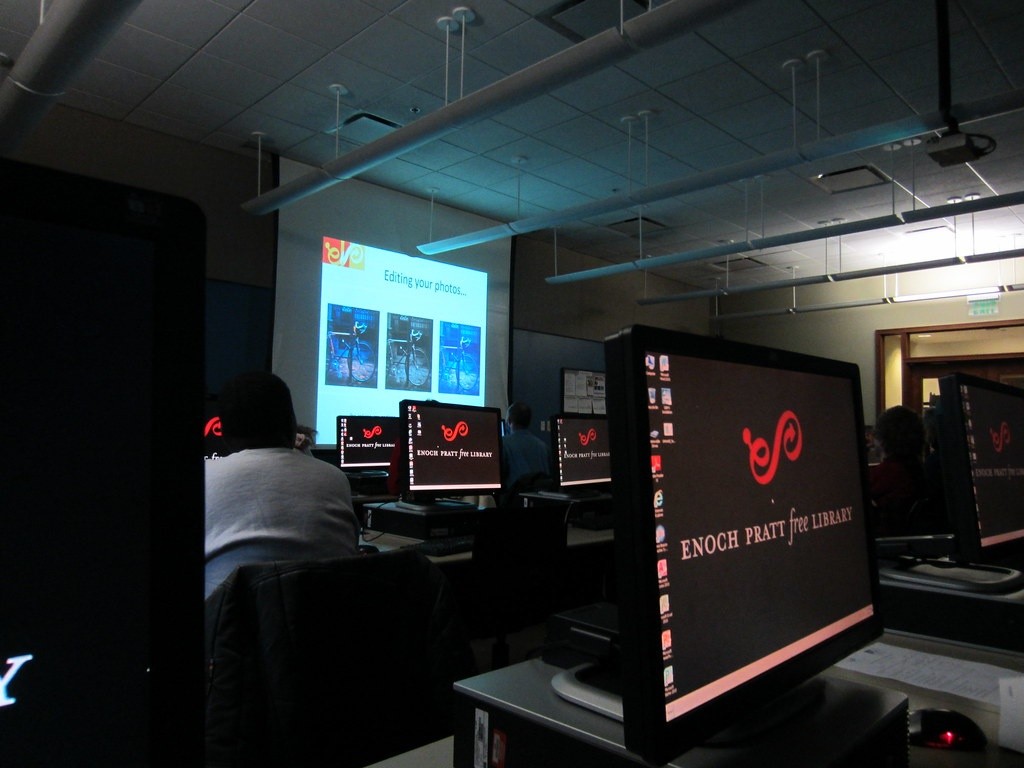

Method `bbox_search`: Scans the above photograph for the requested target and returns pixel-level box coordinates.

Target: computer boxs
[451,652,910,768]
[876,555,1024,657]
[364,502,498,543]
[345,475,391,497]
[517,492,617,530]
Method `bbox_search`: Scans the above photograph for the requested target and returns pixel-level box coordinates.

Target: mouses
[902,707,988,753]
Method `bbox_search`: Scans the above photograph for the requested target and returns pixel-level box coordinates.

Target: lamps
[413,48,1024,322]
[0,0,89,131]
[240,1,761,217]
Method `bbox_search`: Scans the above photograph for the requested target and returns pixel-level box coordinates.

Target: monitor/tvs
[204,394,236,458]
[0,156,213,768]
[337,324,1024,768]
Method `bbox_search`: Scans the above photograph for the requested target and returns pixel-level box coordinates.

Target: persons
[865,403,938,582]
[202,374,366,687]
[500,404,552,497]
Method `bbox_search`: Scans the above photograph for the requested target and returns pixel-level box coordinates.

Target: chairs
[200,497,655,768]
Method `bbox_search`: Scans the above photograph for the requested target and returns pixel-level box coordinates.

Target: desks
[416,522,1024,768]
